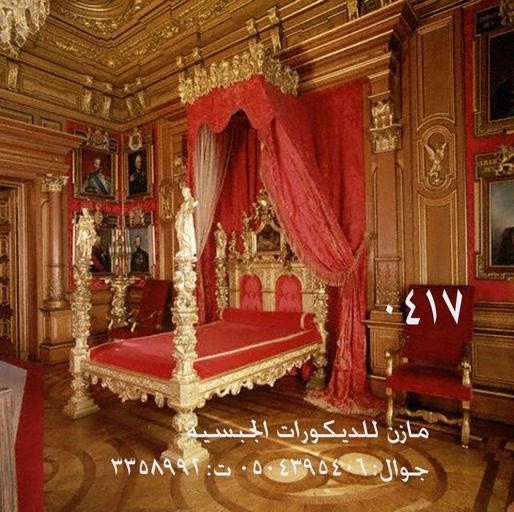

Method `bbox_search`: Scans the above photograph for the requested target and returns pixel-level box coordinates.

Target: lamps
[0,0,50,58]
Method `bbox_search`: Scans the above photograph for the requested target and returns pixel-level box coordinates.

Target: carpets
[0,353,45,512]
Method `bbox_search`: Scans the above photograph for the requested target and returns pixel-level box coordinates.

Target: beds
[62,187,329,472]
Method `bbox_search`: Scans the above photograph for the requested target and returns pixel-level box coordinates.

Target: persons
[128,154,146,193]
[131,235,148,271]
[91,234,108,272]
[82,157,108,194]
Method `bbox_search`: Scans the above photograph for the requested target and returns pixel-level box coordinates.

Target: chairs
[103,280,170,342]
[384,285,474,448]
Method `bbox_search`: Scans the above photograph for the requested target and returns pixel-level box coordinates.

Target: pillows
[220,308,316,330]
